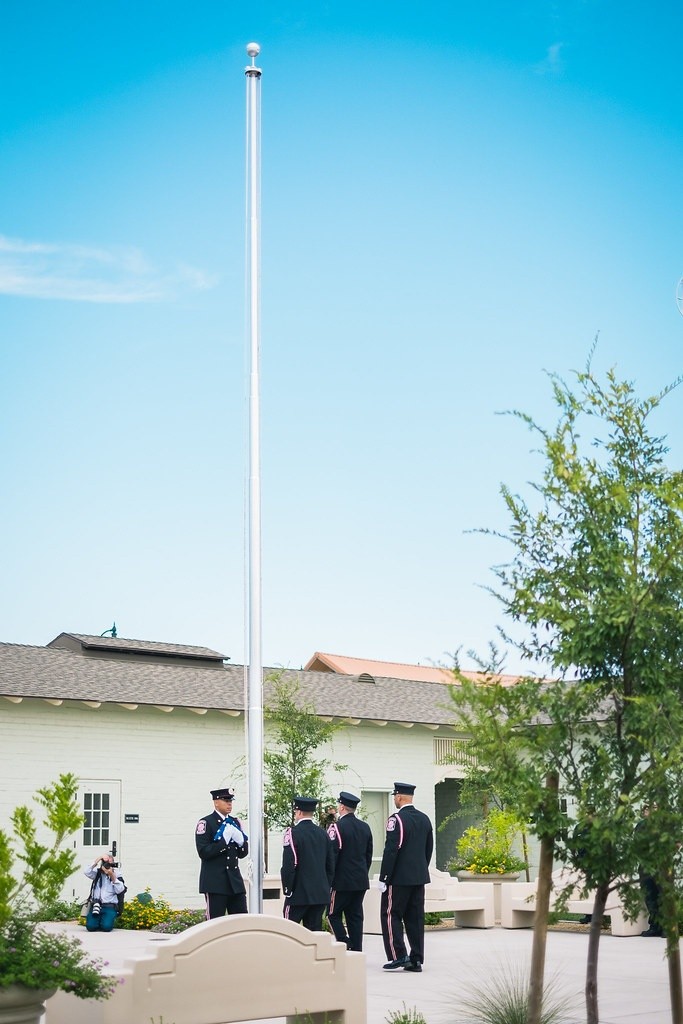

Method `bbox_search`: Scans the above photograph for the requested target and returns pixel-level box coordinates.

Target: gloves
[222,822,244,848]
[379,881,387,893]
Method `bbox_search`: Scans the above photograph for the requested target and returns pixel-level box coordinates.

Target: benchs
[39,914,366,1024]
[500,883,649,936]
[361,868,495,933]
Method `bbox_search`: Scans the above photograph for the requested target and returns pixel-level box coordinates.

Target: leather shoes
[383,955,412,968]
[404,961,422,972]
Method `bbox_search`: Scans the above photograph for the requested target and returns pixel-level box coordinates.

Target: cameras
[91,898,102,916]
[102,861,118,869]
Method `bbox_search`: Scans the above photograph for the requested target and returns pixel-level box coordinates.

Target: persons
[196,787,248,920]
[378,782,433,972]
[572,804,595,923]
[84,855,124,932]
[634,804,681,938]
[281,797,334,931]
[327,792,373,952]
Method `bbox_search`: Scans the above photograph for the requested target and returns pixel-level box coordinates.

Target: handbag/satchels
[80,896,93,917]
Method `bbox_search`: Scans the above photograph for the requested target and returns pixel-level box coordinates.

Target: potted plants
[444,808,532,916]
[0,763,126,1024]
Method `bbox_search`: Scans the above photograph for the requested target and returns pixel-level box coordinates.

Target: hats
[293,797,319,811]
[337,792,360,809]
[391,782,416,795]
[210,788,234,800]
